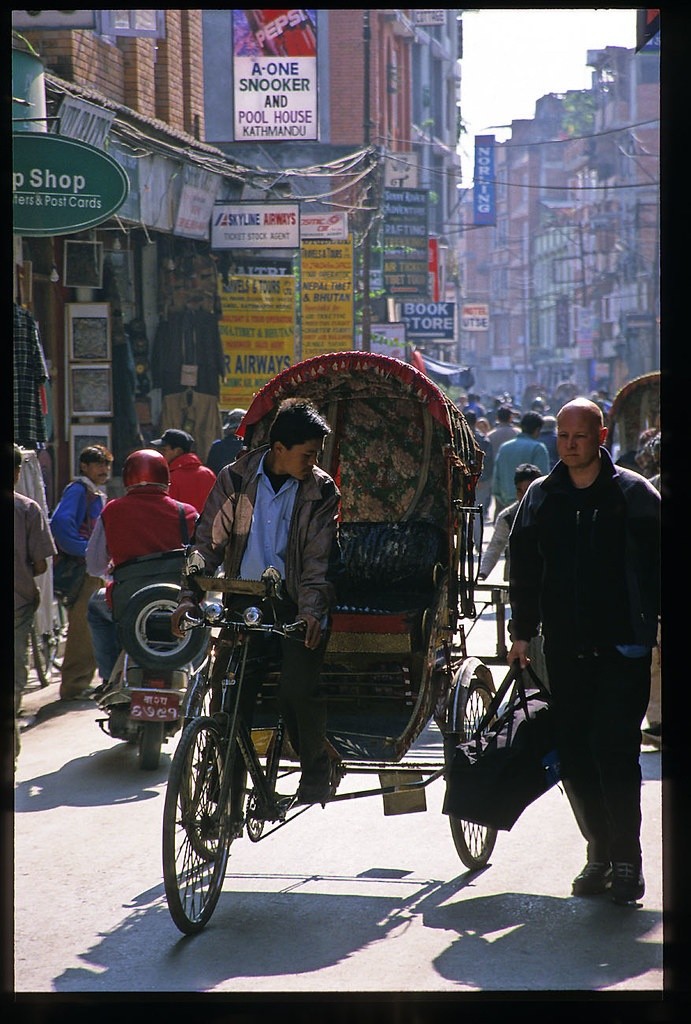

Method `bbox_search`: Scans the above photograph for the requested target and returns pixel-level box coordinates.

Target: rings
[320,633,323,637]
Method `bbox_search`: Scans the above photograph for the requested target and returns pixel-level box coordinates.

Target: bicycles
[29,588,70,686]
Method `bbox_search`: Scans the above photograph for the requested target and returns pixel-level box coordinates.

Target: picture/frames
[69,422,113,481]
[62,240,103,289]
[66,302,112,362]
[68,363,114,417]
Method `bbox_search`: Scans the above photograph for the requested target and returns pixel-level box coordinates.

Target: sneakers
[609,857,646,907]
[571,853,613,894]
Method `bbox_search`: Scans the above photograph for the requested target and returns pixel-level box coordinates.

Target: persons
[50,445,114,700]
[172,397,345,805]
[86,449,212,694]
[508,396,661,905]
[14,443,58,771]
[150,407,248,515]
[454,385,661,582]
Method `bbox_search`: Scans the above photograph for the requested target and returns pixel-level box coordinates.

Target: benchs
[317,521,448,657]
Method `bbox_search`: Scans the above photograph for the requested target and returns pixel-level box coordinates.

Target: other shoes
[640,723,662,750]
[71,687,97,699]
[298,750,338,803]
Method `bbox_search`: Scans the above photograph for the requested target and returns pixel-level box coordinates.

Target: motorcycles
[96,543,214,772]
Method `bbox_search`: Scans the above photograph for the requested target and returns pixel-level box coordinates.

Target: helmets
[122,449,172,490]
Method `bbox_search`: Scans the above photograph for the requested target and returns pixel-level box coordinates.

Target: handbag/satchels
[53,548,87,608]
[112,544,190,625]
[440,657,564,831]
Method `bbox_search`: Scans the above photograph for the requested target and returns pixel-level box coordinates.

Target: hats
[222,409,247,430]
[150,429,194,449]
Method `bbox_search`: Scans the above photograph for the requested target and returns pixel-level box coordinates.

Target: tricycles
[161,357,514,937]
